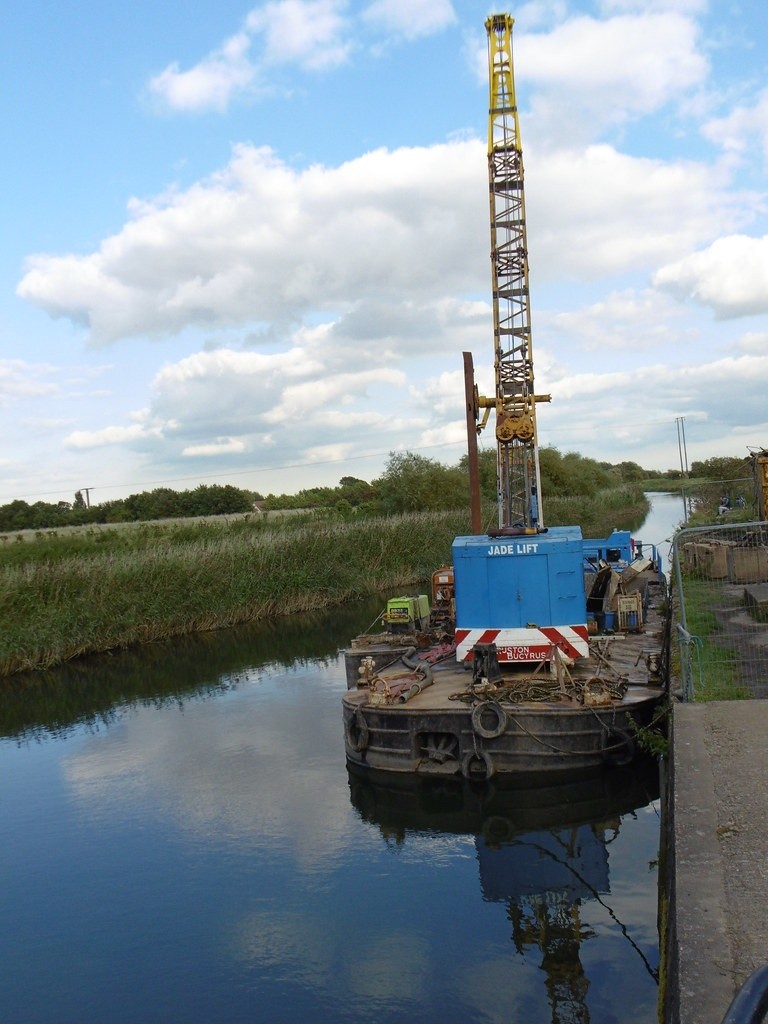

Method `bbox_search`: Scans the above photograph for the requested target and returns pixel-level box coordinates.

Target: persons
[718,496,729,514]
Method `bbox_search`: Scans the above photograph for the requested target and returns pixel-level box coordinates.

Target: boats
[347,780,660,1024]
[342,17,670,780]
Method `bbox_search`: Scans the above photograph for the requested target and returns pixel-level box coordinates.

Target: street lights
[675,418,684,472]
[681,417,689,472]
